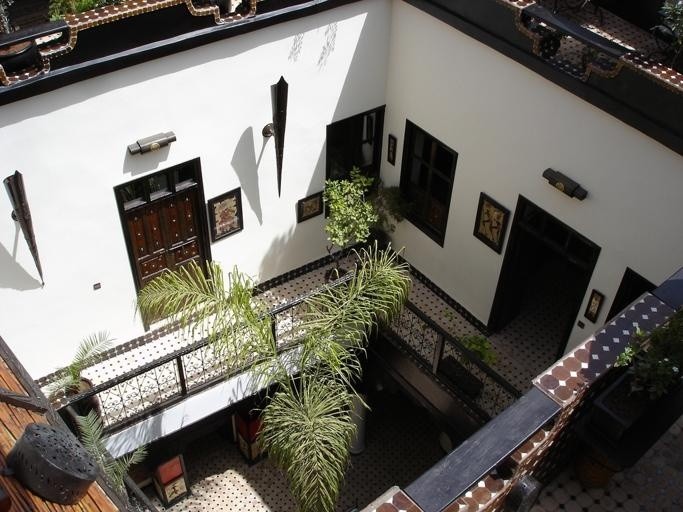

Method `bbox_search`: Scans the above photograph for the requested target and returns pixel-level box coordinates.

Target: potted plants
[423,309,496,400]
[320,165,405,293]
[44,329,117,420]
[590,302,681,446]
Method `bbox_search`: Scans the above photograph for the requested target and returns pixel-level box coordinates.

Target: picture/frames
[584,288,603,324]
[472,192,510,255]
[386,134,396,167]
[297,190,323,223]
[206,186,243,243]
